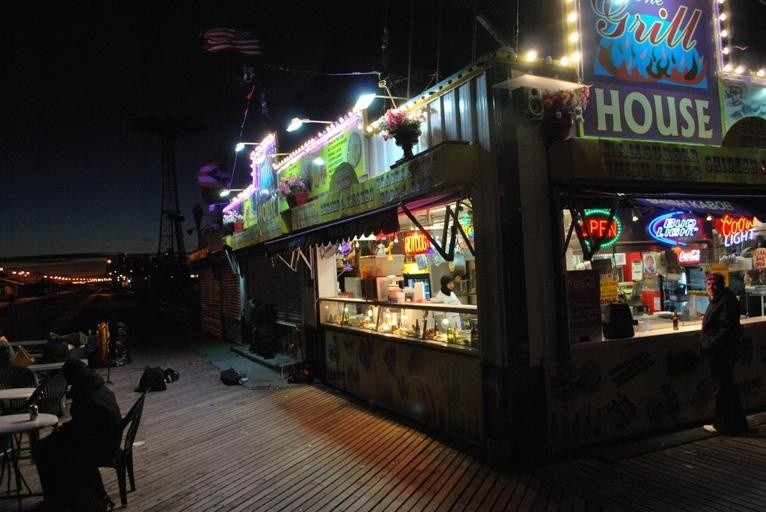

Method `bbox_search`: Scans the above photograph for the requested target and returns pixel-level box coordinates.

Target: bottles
[672,306,680,329]
[342,307,350,325]
[445,318,479,347]
[239,378,248,383]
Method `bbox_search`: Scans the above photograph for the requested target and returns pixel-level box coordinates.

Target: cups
[362,274,426,303]
[29,402,40,421]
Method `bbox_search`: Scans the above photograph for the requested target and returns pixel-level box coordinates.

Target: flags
[199,23,259,57]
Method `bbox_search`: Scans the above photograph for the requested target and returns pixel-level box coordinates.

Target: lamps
[287,116,336,132]
[235,141,260,153]
[219,188,247,198]
[352,93,412,112]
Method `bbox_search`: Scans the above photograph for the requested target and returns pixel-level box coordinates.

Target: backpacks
[136,366,167,393]
[55,464,114,511]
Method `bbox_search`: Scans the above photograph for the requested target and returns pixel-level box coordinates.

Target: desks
[7,340,50,354]
[1,413,59,509]
[28,352,45,358]
[22,358,90,387]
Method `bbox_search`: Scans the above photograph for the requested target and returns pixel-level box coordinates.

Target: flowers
[380,109,422,141]
[542,84,593,122]
[276,176,295,197]
[288,175,306,193]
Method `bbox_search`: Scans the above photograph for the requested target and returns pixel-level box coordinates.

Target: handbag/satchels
[220,367,247,386]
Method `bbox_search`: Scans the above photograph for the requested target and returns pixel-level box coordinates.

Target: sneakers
[703,423,721,433]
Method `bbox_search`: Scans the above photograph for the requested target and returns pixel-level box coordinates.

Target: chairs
[98,385,152,506]
[0,365,39,465]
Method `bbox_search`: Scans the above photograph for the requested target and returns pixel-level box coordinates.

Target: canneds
[28,404,39,421]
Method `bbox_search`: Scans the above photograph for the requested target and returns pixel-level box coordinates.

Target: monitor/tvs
[402,272,432,302]
[601,303,634,338]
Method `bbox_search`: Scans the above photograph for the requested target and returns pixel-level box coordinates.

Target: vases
[286,195,297,208]
[395,134,415,164]
[293,192,307,205]
[548,112,577,141]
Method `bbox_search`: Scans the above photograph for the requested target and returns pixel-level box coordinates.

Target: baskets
[10,345,35,368]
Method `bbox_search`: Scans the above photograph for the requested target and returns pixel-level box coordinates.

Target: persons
[698,273,748,436]
[33,360,123,512]
[432,275,462,334]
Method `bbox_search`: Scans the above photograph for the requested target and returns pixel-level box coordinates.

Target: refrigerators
[680,241,713,293]
[404,272,433,300]
